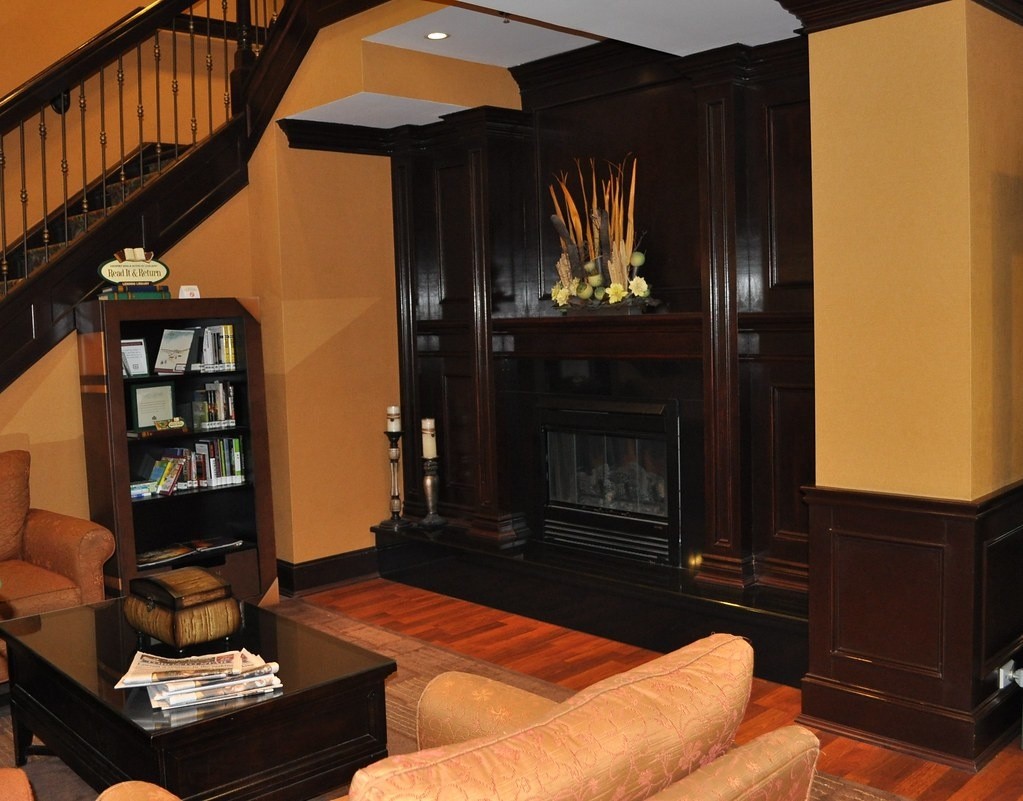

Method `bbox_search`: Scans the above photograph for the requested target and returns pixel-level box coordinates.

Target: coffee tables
[0,596,399,801]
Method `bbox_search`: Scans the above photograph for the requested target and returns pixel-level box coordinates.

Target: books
[97,286,171,300]
[126,325,246,498]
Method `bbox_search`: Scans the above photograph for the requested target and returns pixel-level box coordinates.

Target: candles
[422,418,437,458]
[386,406,401,432]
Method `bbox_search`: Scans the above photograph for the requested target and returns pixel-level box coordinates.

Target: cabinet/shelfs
[76,297,281,608]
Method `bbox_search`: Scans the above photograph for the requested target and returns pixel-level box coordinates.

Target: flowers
[551,275,649,307]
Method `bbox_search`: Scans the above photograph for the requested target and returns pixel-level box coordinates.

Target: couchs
[94,633,820,801]
[0,450,115,683]
